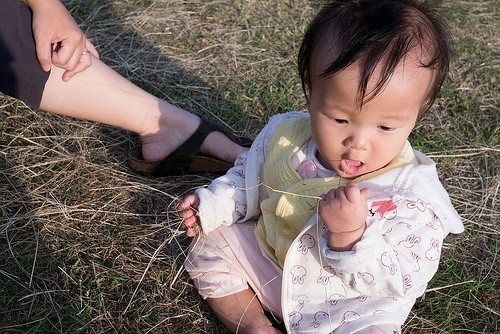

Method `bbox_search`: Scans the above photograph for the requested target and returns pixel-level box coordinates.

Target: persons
[178,1,466,334]
[0,0,254,176]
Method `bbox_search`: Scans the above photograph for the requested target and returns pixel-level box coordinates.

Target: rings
[81,49,90,56]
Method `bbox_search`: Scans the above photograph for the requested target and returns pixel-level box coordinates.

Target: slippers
[128,112,254,175]
